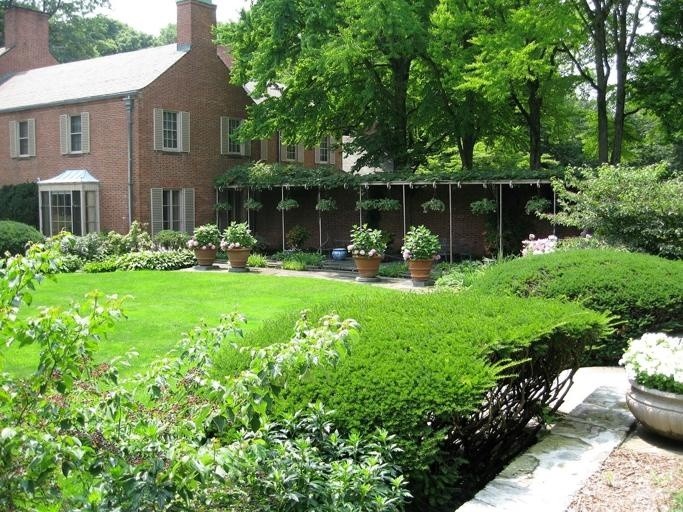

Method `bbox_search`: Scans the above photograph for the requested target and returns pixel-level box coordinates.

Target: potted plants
[283,224,312,258]
[376,228,396,262]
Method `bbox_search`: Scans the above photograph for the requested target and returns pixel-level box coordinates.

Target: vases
[331,248,346,261]
[194,249,216,266]
[225,248,252,268]
[352,255,381,277]
[407,259,432,282]
[624,380,682,442]
[483,240,513,259]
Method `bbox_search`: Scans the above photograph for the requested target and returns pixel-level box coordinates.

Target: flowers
[616,331,682,395]
[399,224,443,262]
[519,234,557,256]
[480,220,513,244]
[219,220,257,252]
[185,223,221,250]
[346,222,387,257]
